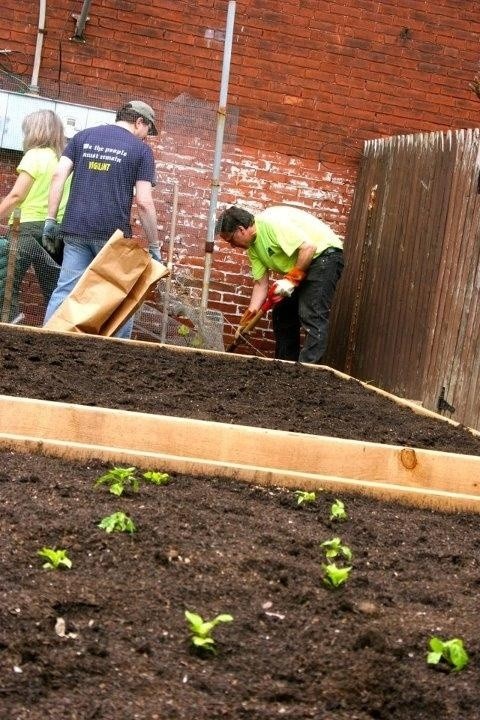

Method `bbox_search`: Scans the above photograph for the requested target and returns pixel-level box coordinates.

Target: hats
[125,100,159,136]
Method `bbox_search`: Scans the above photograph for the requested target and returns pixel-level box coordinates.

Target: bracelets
[283,268,306,287]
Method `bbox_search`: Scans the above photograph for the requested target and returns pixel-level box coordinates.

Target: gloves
[43,217,60,255]
[233,309,258,346]
[149,246,161,262]
[268,268,307,302]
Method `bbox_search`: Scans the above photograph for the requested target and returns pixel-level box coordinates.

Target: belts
[328,247,342,253]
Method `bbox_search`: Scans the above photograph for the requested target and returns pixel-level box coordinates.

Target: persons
[215,206,345,366]
[1,110,72,328]
[41,100,162,340]
